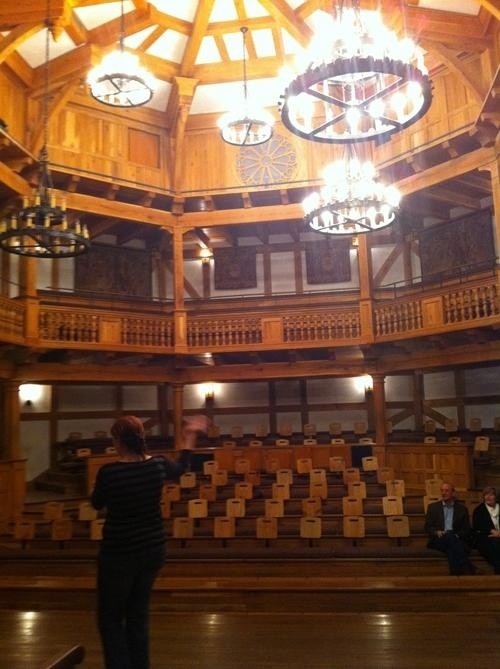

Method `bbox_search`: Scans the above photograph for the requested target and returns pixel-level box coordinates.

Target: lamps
[1,2,94,261]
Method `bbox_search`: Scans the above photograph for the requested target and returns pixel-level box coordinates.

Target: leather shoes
[460,562,472,571]
[449,566,457,576]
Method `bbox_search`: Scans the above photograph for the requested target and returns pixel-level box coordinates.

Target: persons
[472,486,500,574]
[91,414,208,668]
[424,483,485,575]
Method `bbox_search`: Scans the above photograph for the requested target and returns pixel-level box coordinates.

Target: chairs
[1,416,500,560]
